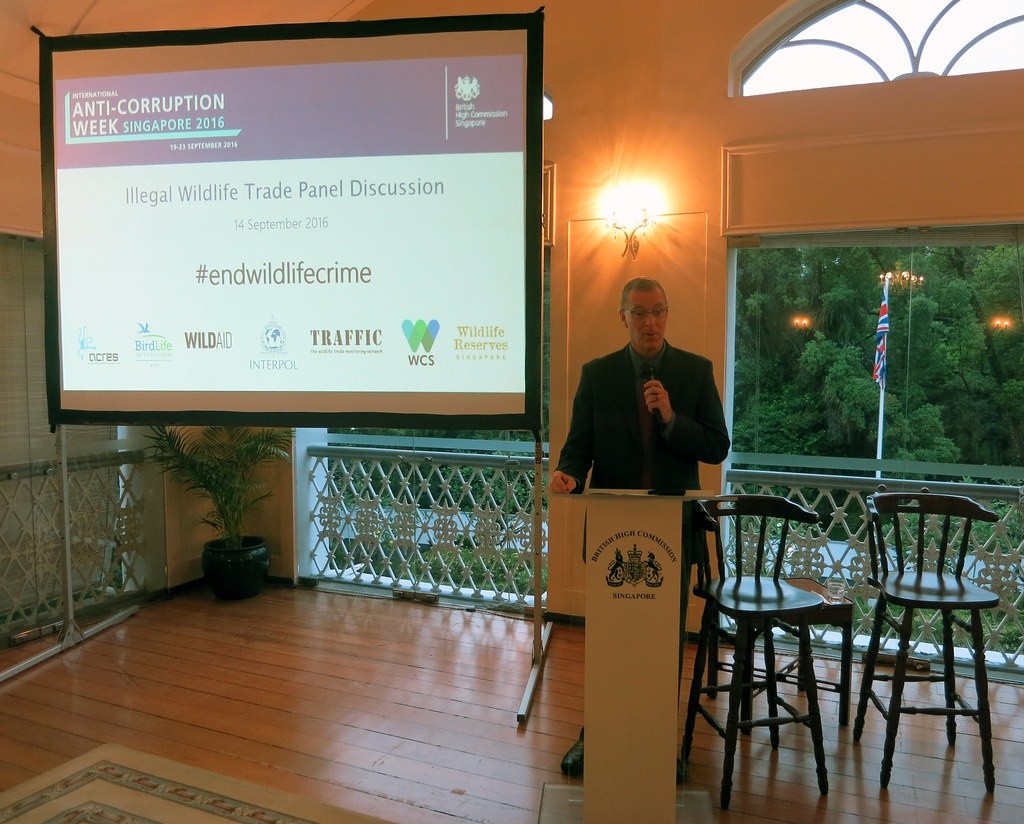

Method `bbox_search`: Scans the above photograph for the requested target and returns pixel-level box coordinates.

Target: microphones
[640,362,662,421]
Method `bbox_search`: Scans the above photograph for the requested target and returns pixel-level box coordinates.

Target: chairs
[847,492,1003,801]
[677,493,830,807]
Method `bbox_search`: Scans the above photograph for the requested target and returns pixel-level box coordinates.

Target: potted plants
[146,424,294,601]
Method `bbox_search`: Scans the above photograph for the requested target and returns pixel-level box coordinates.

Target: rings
[656,394,659,400]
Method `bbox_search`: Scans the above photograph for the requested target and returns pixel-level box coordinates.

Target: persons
[547,278,731,788]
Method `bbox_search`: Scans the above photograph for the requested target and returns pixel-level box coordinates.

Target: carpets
[0,738,416,824]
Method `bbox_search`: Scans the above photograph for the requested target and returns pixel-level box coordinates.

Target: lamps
[599,177,660,261]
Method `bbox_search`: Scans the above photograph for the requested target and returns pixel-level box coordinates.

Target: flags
[873,285,890,390]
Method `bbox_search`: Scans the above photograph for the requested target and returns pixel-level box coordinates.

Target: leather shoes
[562,741,584,776]
[675,761,687,782]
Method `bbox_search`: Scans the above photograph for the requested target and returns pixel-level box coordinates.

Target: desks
[739,573,857,735]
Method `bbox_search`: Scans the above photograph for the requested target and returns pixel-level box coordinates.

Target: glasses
[623,306,668,317]
